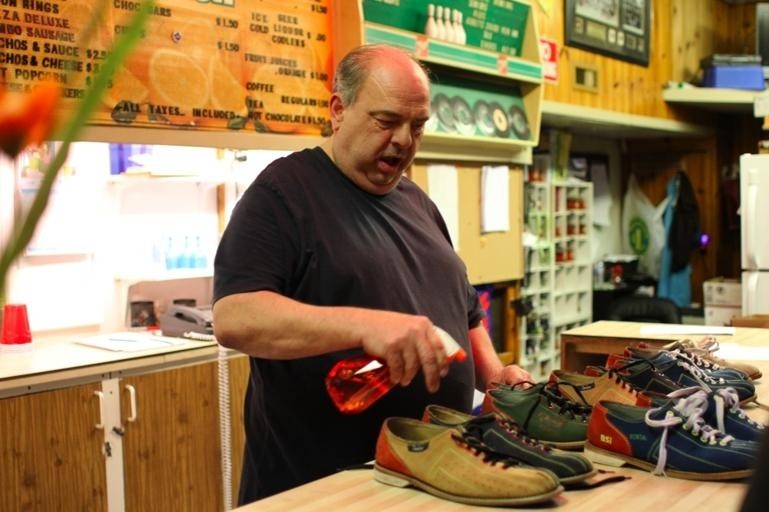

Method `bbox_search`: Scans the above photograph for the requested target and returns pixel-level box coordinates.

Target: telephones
[160,304,213,337]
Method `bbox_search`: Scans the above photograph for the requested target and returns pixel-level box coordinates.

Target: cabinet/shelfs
[0,343,223,512]
[518,182,594,380]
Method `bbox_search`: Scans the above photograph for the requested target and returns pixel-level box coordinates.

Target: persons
[209,39,541,506]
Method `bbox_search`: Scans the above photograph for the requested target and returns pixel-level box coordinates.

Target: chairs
[609,295,681,321]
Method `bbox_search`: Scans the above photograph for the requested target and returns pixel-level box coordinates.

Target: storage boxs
[703,277,743,308]
[703,308,742,327]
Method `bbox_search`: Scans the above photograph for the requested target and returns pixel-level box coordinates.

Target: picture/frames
[563,0,650,68]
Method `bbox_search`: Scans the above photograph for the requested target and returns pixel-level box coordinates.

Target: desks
[560,319,769,375]
[228,359,769,512]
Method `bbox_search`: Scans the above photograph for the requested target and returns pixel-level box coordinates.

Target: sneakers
[659,336,763,382]
[603,342,759,408]
[372,414,565,508]
[479,377,595,450]
[420,401,599,486]
[586,400,769,482]
[635,386,769,442]
[550,365,653,408]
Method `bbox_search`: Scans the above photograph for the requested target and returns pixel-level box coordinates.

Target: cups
[0,302,33,350]
